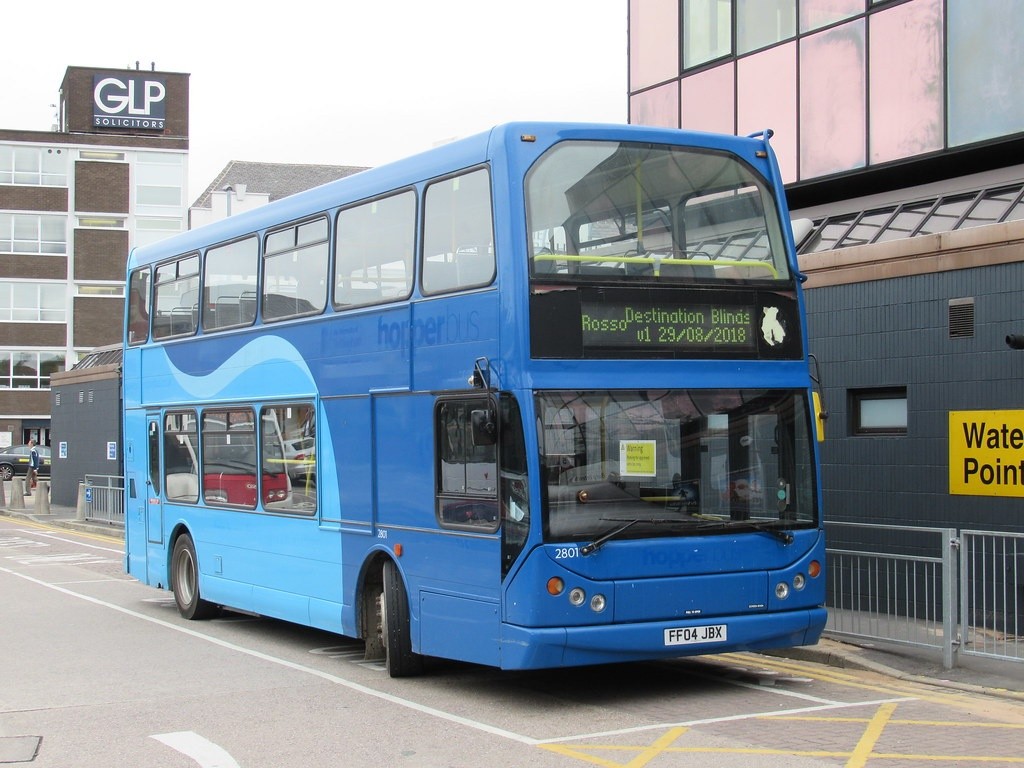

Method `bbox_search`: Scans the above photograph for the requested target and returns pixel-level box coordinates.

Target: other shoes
[47,486,50,493]
[23,493,31,496]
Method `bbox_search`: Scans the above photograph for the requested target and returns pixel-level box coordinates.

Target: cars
[0,444,53,481]
[284,437,318,481]
[149,409,295,510]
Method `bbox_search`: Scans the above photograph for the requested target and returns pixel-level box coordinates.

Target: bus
[120,122,829,681]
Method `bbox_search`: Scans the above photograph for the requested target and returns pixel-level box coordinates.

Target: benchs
[153,244,716,337]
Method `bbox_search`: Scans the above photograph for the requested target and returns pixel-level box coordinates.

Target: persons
[23,439,40,495]
